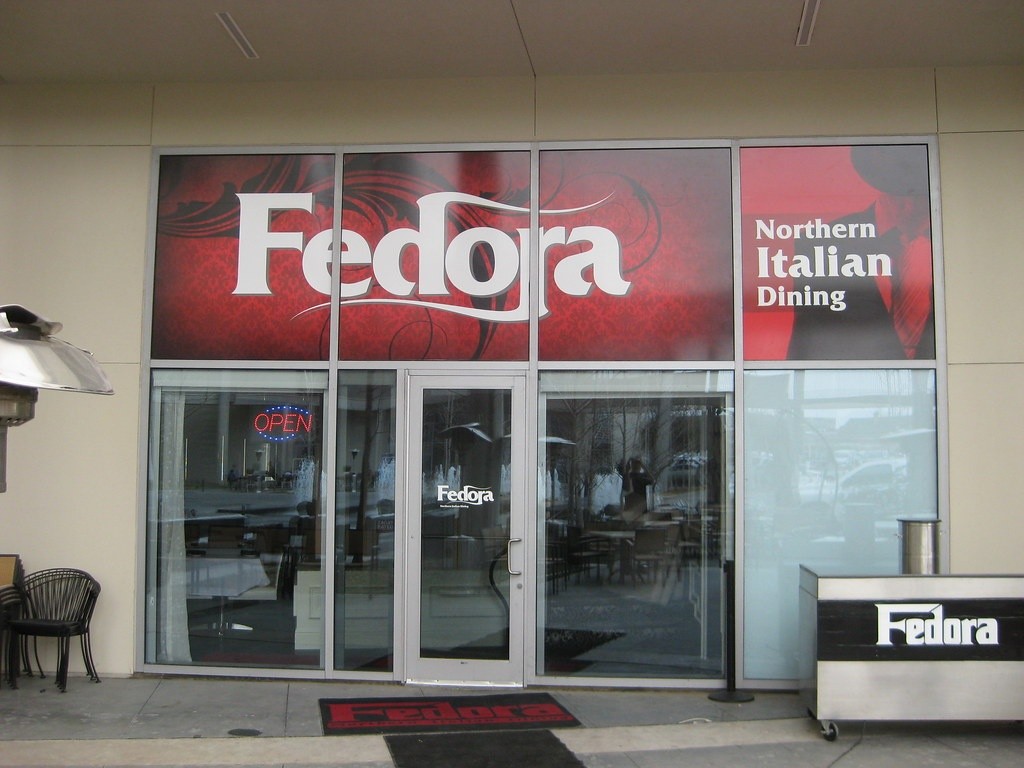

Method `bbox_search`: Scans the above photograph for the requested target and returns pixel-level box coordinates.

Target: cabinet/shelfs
[799,562,1024,739]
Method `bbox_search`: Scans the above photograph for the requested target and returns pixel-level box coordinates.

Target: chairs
[0,554,103,691]
[185,503,718,600]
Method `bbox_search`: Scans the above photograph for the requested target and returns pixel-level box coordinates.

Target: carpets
[382,730,589,768]
[353,622,628,674]
[318,692,582,736]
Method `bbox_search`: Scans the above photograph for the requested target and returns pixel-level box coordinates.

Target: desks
[186,557,271,636]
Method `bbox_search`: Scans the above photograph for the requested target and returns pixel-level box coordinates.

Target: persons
[339,465,356,491]
[618,454,657,517]
